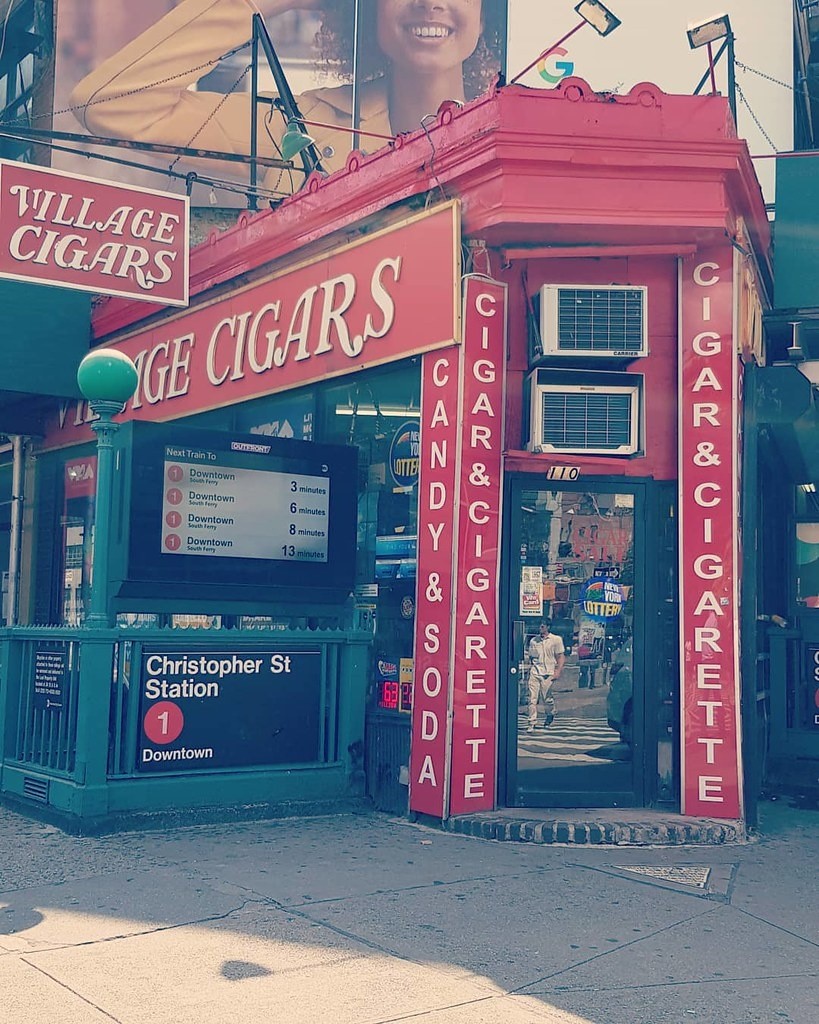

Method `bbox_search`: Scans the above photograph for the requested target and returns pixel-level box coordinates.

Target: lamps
[686,14,732,94]
[333,404,421,417]
[280,117,396,164]
[501,0,621,90]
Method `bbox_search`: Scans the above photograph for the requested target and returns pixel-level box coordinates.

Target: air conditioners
[527,282,650,367]
[535,385,639,455]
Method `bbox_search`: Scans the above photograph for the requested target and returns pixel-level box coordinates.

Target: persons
[591,623,604,652]
[68,1,507,204]
[518,620,565,732]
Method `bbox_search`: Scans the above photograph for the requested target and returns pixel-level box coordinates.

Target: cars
[606,644,634,750]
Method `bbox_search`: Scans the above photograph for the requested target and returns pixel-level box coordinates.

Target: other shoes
[544,713,553,726]
[527,723,535,733]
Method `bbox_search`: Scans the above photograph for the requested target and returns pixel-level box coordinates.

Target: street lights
[77,346,143,631]
